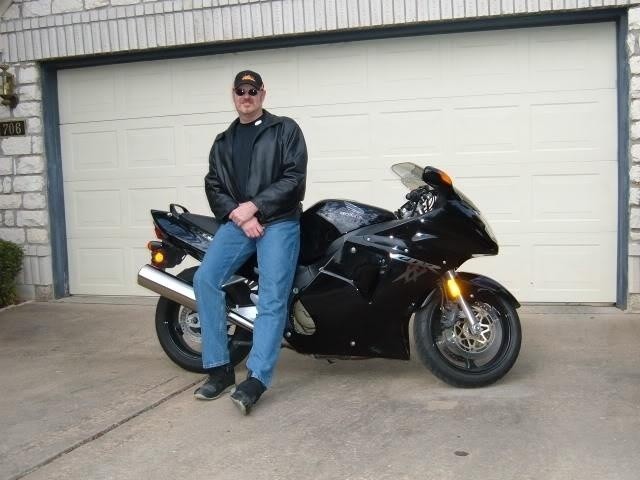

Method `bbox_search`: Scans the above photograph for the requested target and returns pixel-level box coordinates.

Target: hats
[234,70,264,91]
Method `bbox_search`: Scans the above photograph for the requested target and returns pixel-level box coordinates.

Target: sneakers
[194,367,235,399]
[229,371,266,415]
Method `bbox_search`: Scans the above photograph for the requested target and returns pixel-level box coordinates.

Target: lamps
[0,59,17,108]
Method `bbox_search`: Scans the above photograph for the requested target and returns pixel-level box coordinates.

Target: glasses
[235,88,262,96]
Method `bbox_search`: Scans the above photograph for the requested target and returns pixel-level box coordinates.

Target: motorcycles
[138,162,522,389]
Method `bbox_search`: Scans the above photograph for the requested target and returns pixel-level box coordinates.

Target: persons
[193,70,306,417]
